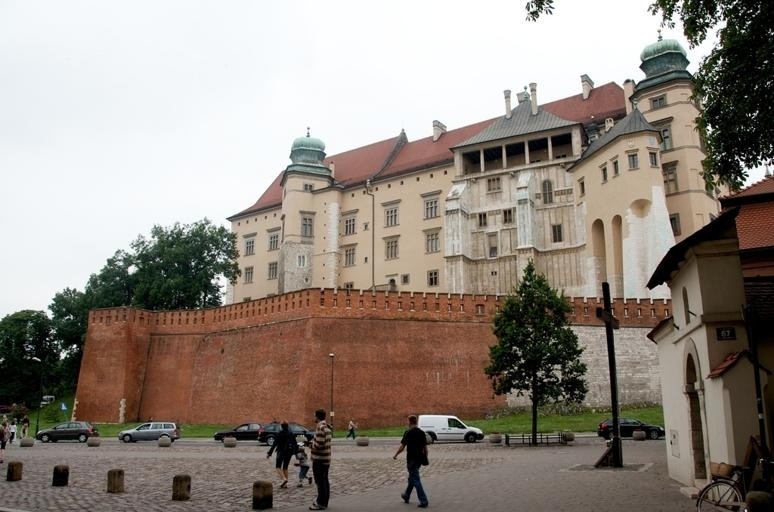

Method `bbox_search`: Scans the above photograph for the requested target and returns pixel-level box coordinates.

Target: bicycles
[694,458,773,511]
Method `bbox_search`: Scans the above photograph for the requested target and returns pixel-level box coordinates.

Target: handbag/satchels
[421,454,429,465]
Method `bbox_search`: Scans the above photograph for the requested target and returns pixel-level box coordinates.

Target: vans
[40,395,54,409]
[117,420,180,443]
[416,414,485,443]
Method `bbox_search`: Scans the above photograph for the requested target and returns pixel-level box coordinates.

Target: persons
[293,441,312,487]
[147,416,153,427]
[392,415,430,507]
[308,408,332,510]
[0,413,30,463]
[266,418,299,488]
[270,417,277,423]
[345,415,359,440]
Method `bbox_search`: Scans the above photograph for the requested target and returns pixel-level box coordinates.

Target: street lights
[31,356,41,433]
[328,352,336,437]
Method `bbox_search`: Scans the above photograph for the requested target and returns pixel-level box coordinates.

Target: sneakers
[278,477,312,489]
[309,504,325,510]
[401,494,409,503]
[418,503,428,508]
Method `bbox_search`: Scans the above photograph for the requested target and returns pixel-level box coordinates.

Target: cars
[596,416,666,441]
[33,420,101,444]
[257,420,315,447]
[212,419,264,444]
